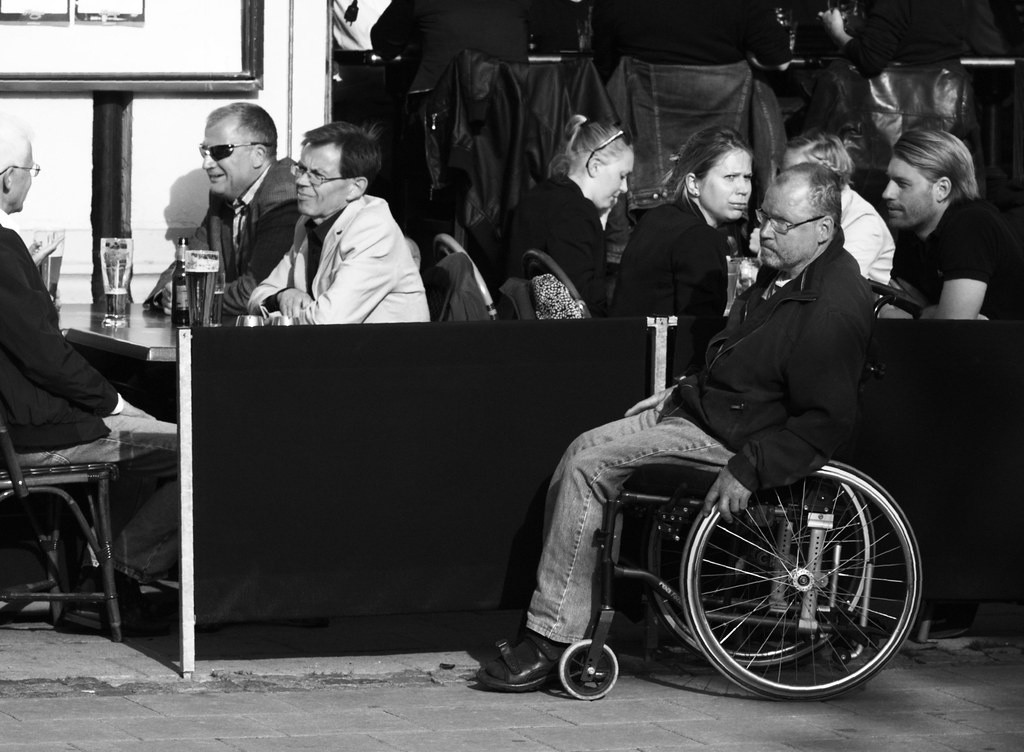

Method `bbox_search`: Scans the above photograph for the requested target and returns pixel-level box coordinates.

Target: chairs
[434,233,498,322]
[520,249,592,318]
[0,424,122,641]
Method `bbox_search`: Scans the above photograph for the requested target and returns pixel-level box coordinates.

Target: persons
[370,0,564,86]
[496,113,634,318]
[475,160,872,691]
[875,128,1024,321]
[749,126,895,298]
[0,125,181,632]
[245,120,431,325]
[142,102,301,316]
[818,0,1014,198]
[607,123,753,316]
[591,0,795,90]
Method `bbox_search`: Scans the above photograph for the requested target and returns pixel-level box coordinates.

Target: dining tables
[56,303,237,362]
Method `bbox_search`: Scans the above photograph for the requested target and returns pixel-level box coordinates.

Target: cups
[272,317,300,326]
[184,250,220,327]
[724,254,760,319]
[100,238,134,327]
[210,272,227,327]
[234,314,265,327]
[33,229,65,302]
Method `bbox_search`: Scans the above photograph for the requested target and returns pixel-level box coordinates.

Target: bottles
[171,238,190,327]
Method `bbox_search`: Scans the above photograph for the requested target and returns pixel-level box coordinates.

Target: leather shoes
[63,585,170,637]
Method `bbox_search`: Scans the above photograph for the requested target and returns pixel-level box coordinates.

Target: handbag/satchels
[799,57,985,198]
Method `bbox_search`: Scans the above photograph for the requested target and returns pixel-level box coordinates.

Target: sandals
[475,632,592,691]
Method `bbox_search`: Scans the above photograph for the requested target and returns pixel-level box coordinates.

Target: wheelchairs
[556,277,925,702]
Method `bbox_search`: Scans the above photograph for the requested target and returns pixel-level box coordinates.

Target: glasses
[586,129,630,168]
[291,163,357,185]
[756,207,837,235]
[0,163,41,175]
[199,142,272,160]
